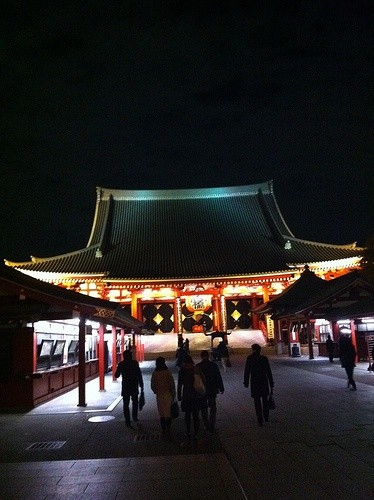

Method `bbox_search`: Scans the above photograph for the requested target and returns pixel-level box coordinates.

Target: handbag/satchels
[139,392,145,411]
[171,396,179,416]
[226,359,232,367]
[268,393,275,410]
[194,367,203,392]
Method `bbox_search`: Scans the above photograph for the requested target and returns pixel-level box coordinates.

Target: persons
[337,339,357,391]
[151,357,176,433]
[243,343,274,423]
[195,350,225,432]
[115,350,144,429]
[217,342,229,373]
[326,335,334,362]
[177,356,204,438]
